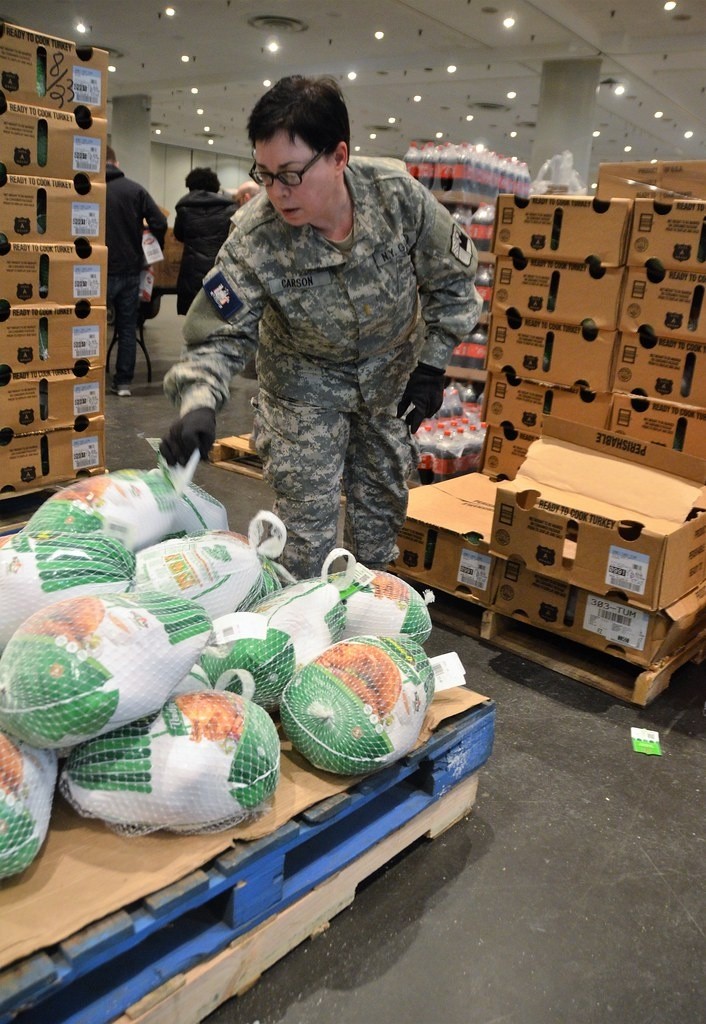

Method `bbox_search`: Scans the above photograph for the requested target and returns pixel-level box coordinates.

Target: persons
[159,73,484,581]
[172,167,261,316]
[106,147,168,397]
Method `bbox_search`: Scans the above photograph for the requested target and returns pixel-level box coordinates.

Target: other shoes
[111,383,130,396]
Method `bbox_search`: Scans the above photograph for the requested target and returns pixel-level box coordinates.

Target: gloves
[397,360,447,435]
[157,406,216,466]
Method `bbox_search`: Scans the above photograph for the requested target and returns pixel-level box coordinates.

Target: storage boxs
[1,21,705,670]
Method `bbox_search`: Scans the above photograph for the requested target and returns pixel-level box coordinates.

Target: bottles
[400,141,531,369]
[410,377,488,485]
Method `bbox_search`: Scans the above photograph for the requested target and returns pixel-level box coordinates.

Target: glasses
[248,143,334,187]
